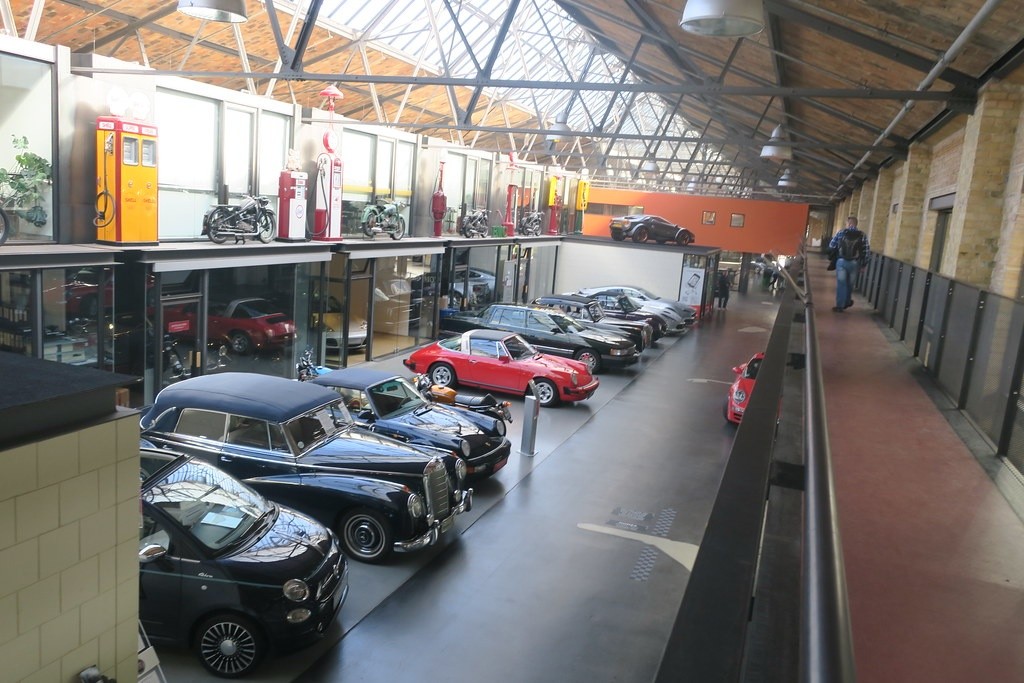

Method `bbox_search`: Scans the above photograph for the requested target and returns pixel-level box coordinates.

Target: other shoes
[832,306,843,313]
[718,307,726,311]
[844,300,853,309]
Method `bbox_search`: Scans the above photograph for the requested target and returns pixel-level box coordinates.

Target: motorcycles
[201,192,277,244]
[292,347,364,400]
[520,210,545,235]
[359,198,410,240]
[413,374,514,424]
[462,209,492,239]
[160,332,187,379]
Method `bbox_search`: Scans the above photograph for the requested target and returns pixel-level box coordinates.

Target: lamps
[778,167,799,186]
[759,125,792,159]
[686,177,698,191]
[644,155,660,174]
[679,0,765,38]
[545,110,573,142]
[177,0,248,23]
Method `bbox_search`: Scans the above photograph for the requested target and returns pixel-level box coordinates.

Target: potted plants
[0,134,54,239]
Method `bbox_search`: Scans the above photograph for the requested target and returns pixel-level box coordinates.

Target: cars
[139,372,475,564]
[438,300,640,374]
[40,268,155,316]
[723,352,782,425]
[408,266,497,322]
[311,366,512,485]
[749,261,763,273]
[311,292,369,353]
[138,447,350,679]
[531,284,699,353]
[165,297,298,354]
[403,329,599,405]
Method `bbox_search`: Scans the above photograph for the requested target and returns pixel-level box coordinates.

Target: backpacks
[842,228,862,260]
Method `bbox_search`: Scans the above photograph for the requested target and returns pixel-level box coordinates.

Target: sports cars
[610,214,695,245]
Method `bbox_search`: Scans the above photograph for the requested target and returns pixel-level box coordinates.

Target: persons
[829,215,871,312]
[718,270,731,310]
[768,267,779,290]
